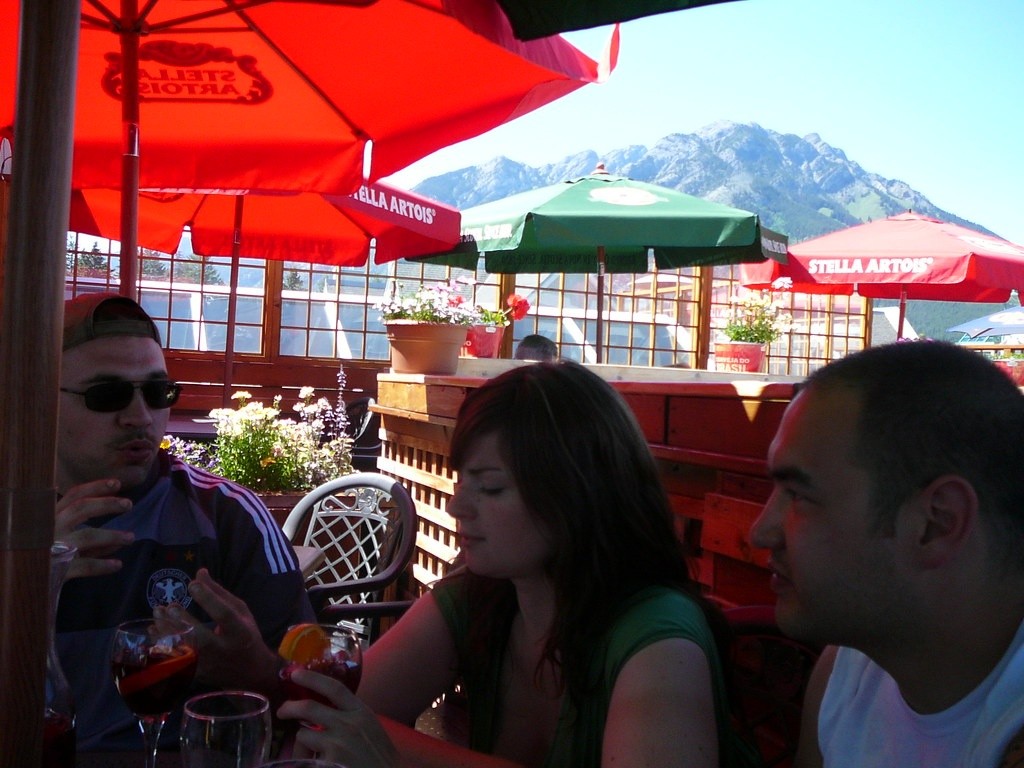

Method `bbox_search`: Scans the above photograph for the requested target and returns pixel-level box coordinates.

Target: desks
[163,408,218,438]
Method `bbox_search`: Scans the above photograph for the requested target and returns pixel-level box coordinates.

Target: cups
[274,624,363,730]
[177,690,346,768]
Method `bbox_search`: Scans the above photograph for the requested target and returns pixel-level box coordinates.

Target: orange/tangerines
[279,623,330,661]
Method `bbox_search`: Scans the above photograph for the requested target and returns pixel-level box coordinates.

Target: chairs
[717,599,817,767]
[281,469,427,696]
[322,395,384,474]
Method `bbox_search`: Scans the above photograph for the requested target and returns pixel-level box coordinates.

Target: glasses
[56,382,184,414]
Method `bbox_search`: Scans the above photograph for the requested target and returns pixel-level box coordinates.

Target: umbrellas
[65,176,460,415]
[0,0,602,296]
[404,160,789,364]
[736,206,1024,344]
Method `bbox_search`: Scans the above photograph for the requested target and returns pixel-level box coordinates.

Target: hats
[60,291,162,354]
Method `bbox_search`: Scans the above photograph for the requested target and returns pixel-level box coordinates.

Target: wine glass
[108,617,206,768]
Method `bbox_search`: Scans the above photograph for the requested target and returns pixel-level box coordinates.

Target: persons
[37,290,319,755]
[272,358,739,768]
[744,341,1024,766]
[514,334,559,360]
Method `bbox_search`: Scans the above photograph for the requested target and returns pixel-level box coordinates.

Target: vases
[256,492,362,587]
[467,325,507,359]
[715,344,763,371]
[383,318,469,375]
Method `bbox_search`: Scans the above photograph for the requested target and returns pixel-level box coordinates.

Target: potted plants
[991,353,1024,387]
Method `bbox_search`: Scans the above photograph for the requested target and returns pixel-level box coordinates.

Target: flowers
[726,277,794,344]
[161,362,363,493]
[381,278,470,326]
[465,293,530,328]
